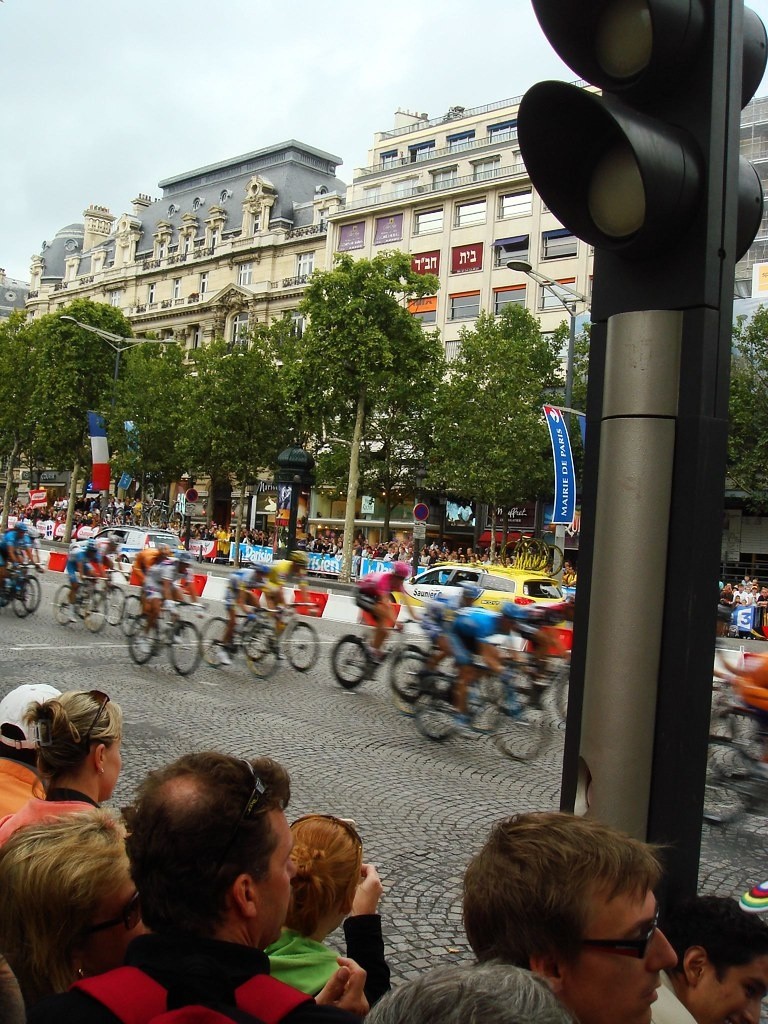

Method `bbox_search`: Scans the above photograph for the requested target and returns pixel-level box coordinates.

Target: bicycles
[238,597,321,673]
[327,617,571,763]
[73,568,126,626]
[137,498,185,533]
[50,573,113,634]
[198,604,289,681]
[124,599,205,676]
[500,526,566,578]
[115,582,193,646]
[702,678,768,829]
[0,560,47,619]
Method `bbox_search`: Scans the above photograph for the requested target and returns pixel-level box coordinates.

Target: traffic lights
[715,0,768,421]
[517,0,730,324]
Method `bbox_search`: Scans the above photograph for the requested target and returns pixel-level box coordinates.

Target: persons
[353,557,420,658]
[25,750,370,1024]
[214,565,283,666]
[259,550,311,634]
[0,683,61,820]
[0,688,130,856]
[131,541,173,585]
[64,534,126,623]
[134,556,199,654]
[363,960,579,1024]
[648,894,768,1024]
[462,811,678,1024]
[0,495,516,570]
[713,573,768,780]
[258,812,392,1011]
[0,523,45,609]
[417,579,574,736]
[0,820,151,1024]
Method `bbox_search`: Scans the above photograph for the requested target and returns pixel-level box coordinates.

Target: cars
[67,523,192,569]
[399,560,569,628]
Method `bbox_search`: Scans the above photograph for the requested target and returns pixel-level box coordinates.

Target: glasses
[85,688,110,746]
[289,813,362,871]
[66,890,143,944]
[236,758,275,860]
[561,902,659,959]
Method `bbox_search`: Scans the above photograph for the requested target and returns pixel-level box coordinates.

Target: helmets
[254,562,273,573]
[290,550,309,565]
[15,523,28,531]
[27,526,39,538]
[108,535,122,547]
[462,584,484,600]
[84,540,97,549]
[156,542,172,556]
[501,602,529,618]
[175,549,195,564]
[393,561,411,576]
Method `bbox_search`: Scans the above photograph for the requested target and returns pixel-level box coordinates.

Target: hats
[0,684,64,750]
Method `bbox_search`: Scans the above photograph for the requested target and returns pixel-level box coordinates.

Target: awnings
[476,531,540,551]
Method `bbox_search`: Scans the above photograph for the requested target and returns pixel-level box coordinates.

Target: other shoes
[140,642,151,654]
[219,651,232,665]
[69,609,76,623]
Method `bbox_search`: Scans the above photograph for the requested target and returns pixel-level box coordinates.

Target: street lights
[503,257,589,591]
[58,312,180,514]
